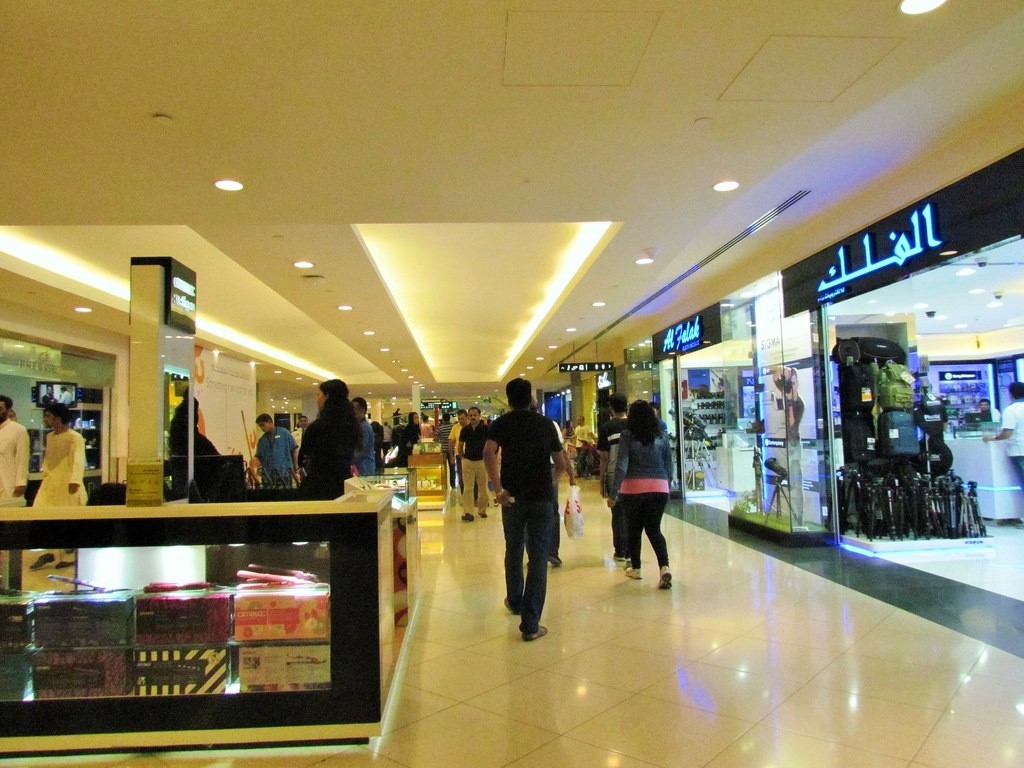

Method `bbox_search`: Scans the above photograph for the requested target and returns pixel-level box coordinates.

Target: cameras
[776,375,792,393]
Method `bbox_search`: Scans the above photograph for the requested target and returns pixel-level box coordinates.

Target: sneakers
[624,566,641,579]
[658,568,672,589]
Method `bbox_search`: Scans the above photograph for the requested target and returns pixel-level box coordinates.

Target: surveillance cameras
[994,292,1003,299]
[975,257,988,268]
[925,311,936,318]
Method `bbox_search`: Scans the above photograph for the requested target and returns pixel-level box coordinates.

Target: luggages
[837,336,920,466]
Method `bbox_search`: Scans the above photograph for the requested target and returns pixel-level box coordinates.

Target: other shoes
[479,511,487,519]
[614,552,624,559]
[462,514,476,520]
[523,625,547,641]
[548,554,561,566]
[56,559,75,570]
[625,560,631,570]
[28,553,54,569]
[505,596,522,615]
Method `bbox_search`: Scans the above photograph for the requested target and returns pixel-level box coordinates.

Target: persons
[289,414,309,484]
[250,414,301,489]
[562,419,575,452]
[482,377,578,641]
[29,402,89,570]
[978,399,1002,428]
[419,414,435,438]
[371,408,421,476]
[297,379,365,502]
[574,415,598,478]
[710,368,731,391]
[984,382,1024,531]
[347,394,375,477]
[448,407,502,522]
[596,392,675,589]
[0,394,31,508]
[433,412,457,489]
[773,362,805,527]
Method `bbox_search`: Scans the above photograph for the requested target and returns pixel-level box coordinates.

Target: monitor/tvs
[36,380,77,409]
[965,412,991,423]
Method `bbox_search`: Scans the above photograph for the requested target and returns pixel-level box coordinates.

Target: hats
[606,393,628,407]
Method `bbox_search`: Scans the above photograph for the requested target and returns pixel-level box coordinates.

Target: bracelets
[993,434,997,440]
[496,488,504,495]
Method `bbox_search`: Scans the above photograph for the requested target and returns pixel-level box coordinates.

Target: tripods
[684,427,719,492]
[764,476,798,524]
[836,464,987,542]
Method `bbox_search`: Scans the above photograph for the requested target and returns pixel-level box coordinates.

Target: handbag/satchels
[564,487,584,538]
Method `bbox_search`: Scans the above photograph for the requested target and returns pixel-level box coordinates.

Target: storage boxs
[132,647,234,695]
[239,639,334,694]
[33,589,135,647]
[136,586,234,644]
[0,592,38,645]
[234,576,326,642]
[0,648,34,701]
[32,646,130,699]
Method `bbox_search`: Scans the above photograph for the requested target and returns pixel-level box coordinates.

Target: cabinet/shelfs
[692,398,726,416]
